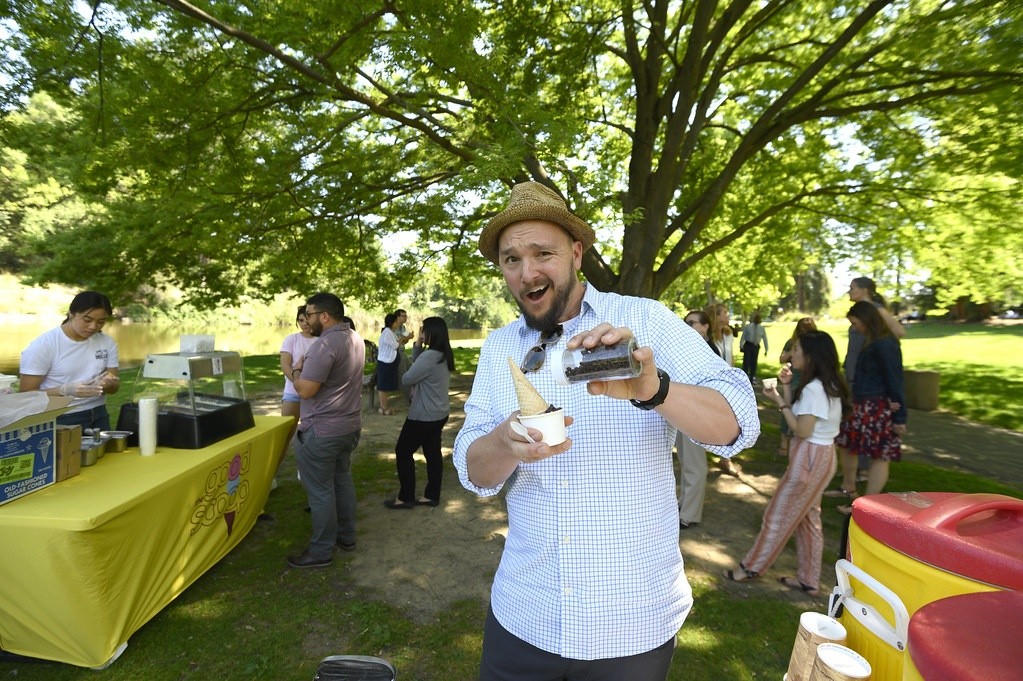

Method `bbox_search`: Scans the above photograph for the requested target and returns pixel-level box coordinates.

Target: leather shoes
[336,539,357,550]
[286,549,333,568]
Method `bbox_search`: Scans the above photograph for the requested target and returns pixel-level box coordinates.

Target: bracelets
[292,369,302,376]
[781,382,791,385]
[629,367,670,411]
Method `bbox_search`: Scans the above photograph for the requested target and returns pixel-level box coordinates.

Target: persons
[780,317,817,458]
[821,276,908,516]
[739,315,768,385]
[376,310,415,415]
[721,331,843,594]
[452,181,760,681]
[384,317,455,510]
[269,292,366,566]
[676,303,733,530]
[20,291,120,436]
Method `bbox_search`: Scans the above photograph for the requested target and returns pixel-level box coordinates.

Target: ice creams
[507,355,561,416]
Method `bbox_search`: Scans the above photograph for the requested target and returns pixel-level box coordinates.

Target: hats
[478,181,596,263]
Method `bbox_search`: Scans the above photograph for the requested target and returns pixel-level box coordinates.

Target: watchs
[778,405,791,412]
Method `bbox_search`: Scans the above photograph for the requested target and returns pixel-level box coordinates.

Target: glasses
[297,306,303,311]
[305,311,324,319]
[519,322,564,374]
[688,320,701,327]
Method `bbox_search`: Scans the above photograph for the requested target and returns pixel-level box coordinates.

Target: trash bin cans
[903,370,941,411]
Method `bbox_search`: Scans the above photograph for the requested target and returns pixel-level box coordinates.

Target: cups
[783,612,872,681]
[562,338,642,385]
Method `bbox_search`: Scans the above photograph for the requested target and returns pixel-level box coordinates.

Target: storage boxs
[0,396,83,506]
[836,492,1023,681]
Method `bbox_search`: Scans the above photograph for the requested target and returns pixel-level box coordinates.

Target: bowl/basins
[762,378,777,390]
[516,409,566,446]
[138,398,157,455]
[81,431,134,466]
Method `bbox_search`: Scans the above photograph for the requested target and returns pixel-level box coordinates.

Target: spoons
[510,421,535,444]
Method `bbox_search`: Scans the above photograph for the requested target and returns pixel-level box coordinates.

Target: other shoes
[680,520,696,529]
[415,497,439,506]
[719,459,739,475]
[384,499,415,509]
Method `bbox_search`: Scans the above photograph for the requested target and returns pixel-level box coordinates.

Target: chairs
[363,339,382,407]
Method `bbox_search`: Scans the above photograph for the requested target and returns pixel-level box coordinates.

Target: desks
[0,415,297,669]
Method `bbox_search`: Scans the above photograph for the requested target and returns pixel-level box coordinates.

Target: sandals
[778,575,818,597]
[840,486,860,499]
[721,563,762,581]
[377,407,396,415]
